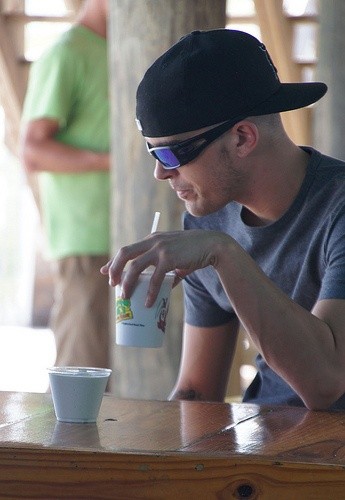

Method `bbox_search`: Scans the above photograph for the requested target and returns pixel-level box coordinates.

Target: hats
[134,28,329,139]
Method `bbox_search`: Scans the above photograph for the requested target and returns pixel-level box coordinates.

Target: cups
[115,272,175,348]
[48,367,112,424]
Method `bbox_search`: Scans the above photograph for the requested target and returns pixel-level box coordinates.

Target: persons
[17,0,126,392]
[179,402,340,456]
[100,29,345,413]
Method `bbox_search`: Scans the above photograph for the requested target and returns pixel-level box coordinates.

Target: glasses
[145,115,243,169]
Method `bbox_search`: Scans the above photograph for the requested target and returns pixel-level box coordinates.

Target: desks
[0,390,344,500]
